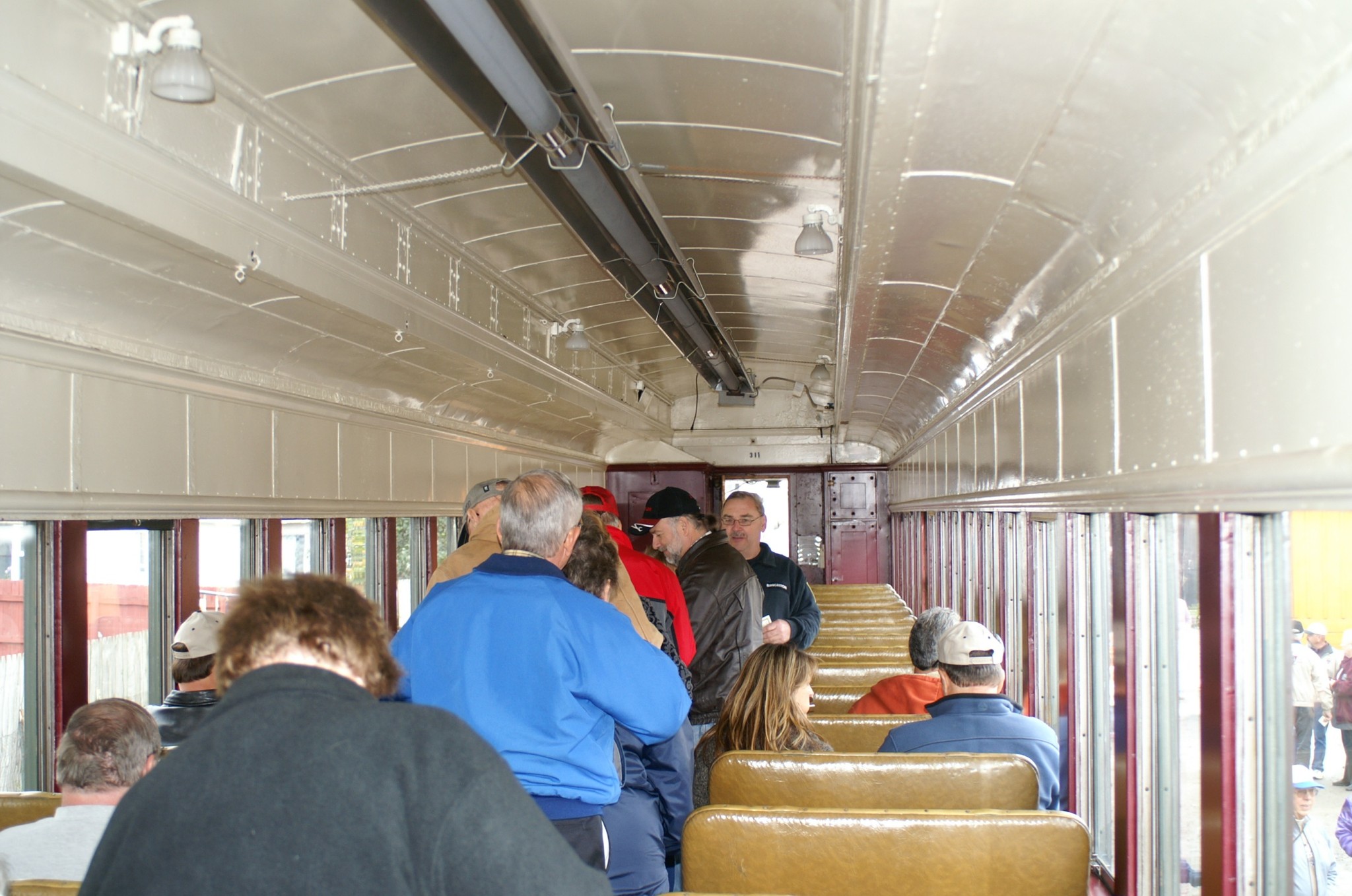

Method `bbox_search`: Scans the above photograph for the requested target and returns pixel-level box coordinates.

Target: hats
[1292,621,1303,633]
[579,486,619,516]
[1290,766,1325,789]
[456,478,512,548]
[937,621,1004,665]
[631,487,701,535]
[1304,623,1327,635]
[171,611,228,658]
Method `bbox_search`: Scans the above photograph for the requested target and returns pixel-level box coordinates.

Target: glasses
[1294,789,1319,798]
[722,516,762,526]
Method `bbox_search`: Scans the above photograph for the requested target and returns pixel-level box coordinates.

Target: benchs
[660,583,1092,896]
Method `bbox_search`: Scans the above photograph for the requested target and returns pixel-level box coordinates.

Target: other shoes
[1346,784,1352,791]
[1313,769,1324,779]
[1333,778,1352,785]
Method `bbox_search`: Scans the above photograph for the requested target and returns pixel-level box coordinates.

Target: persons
[1289,620,1352,896]
[846,608,961,714]
[381,469,833,896]
[140,610,223,746]
[0,698,163,896]
[76,572,612,896]
[876,621,1060,810]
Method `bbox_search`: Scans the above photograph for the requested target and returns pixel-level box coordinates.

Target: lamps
[111,14,215,103]
[810,355,836,379]
[636,381,654,413]
[551,318,591,351]
[795,204,844,255]
